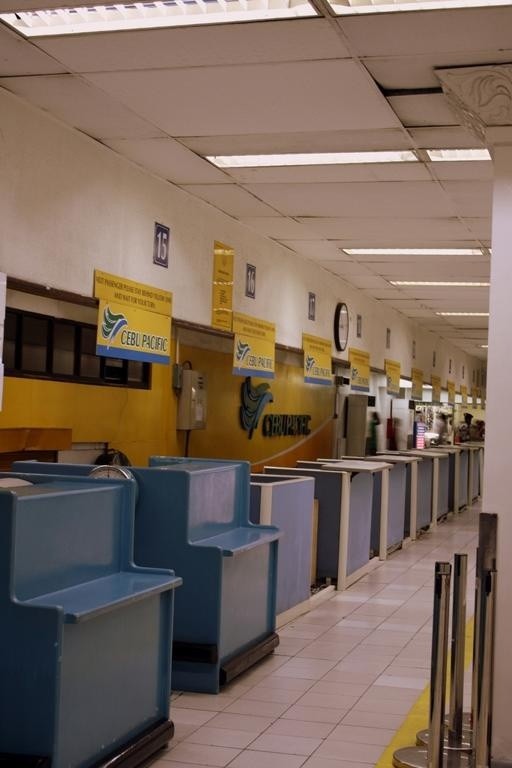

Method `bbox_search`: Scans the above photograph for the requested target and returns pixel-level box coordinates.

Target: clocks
[334,303,350,350]
